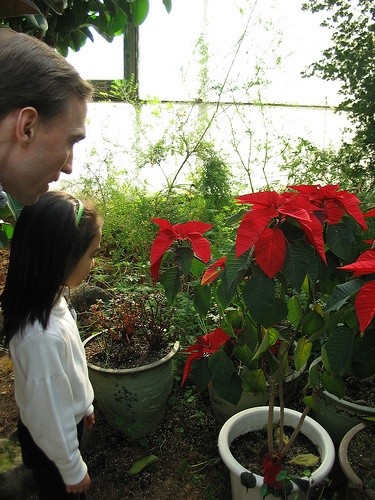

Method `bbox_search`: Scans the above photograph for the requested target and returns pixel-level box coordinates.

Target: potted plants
[202,189,311,421]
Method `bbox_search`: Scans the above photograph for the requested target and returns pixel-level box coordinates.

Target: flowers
[89,290,170,367]
[148,184,375,500]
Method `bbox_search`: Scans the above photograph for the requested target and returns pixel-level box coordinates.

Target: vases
[218,406,336,500]
[337,419,375,500]
[82,325,181,439]
[308,356,375,444]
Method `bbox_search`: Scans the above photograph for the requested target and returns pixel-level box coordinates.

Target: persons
[0,30,93,207]
[0,192,102,500]
